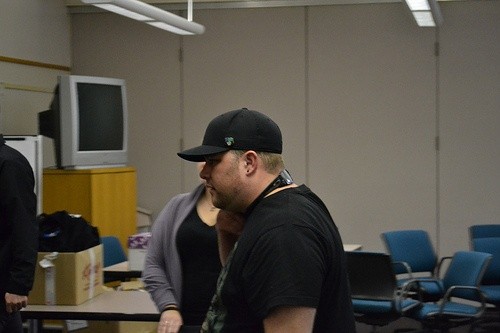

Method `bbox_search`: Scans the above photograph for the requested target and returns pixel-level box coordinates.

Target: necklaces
[211,207,215,209]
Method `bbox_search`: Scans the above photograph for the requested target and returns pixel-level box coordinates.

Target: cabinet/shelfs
[42,167,138,288]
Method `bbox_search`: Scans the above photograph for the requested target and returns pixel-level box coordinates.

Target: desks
[102,260,143,283]
[19,288,162,333]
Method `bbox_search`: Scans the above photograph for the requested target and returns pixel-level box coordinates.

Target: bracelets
[161,307,182,314]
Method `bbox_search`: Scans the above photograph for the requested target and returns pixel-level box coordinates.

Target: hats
[177,108,283,163]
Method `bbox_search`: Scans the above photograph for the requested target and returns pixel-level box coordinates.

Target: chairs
[100,236,128,268]
[344,224,500,333]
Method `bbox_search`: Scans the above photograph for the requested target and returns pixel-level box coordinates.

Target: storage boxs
[27,244,104,307]
[128,233,152,271]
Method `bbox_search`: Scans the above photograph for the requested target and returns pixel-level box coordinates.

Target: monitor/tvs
[36,75,129,170]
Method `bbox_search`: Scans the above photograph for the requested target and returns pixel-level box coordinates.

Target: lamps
[403,0,443,28]
[84,0,205,36]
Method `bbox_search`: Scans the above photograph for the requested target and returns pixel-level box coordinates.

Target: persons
[0,133,37,333]
[177,108,356,333]
[141,163,221,333]
